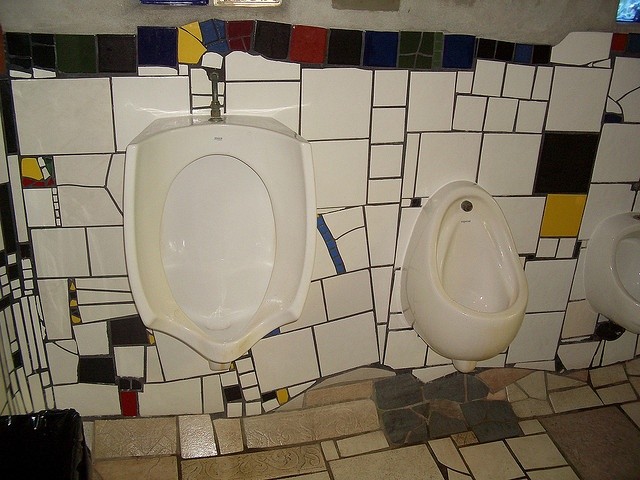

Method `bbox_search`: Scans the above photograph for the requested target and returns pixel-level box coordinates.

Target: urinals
[400,179,530,373]
[123,117,317,371]
[586,211,640,334]
